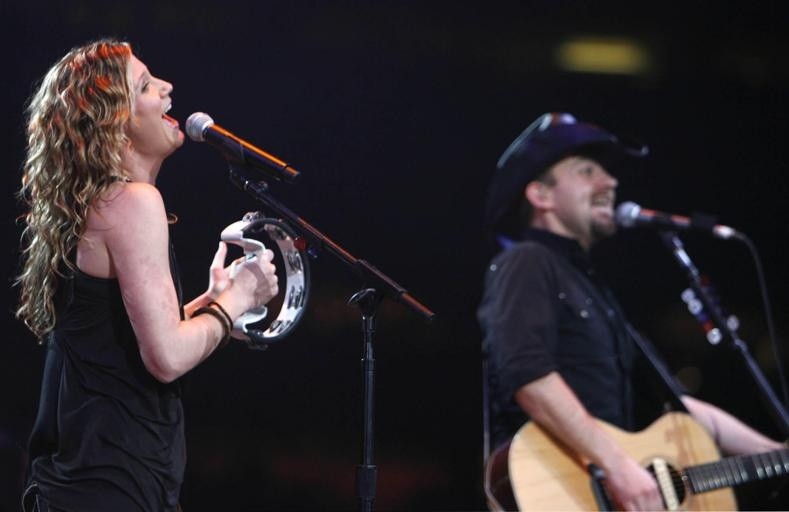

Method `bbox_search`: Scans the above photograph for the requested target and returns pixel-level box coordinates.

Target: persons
[16,39,280,511]
[478,110,788,511]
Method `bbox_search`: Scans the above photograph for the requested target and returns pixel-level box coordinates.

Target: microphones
[615,201,736,240]
[185,111,301,184]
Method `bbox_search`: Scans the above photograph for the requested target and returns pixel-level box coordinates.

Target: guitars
[484,412,788,510]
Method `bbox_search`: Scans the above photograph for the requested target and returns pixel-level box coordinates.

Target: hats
[492,112,619,221]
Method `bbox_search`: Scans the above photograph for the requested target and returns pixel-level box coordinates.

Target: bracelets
[207,300,235,331]
[191,307,230,353]
